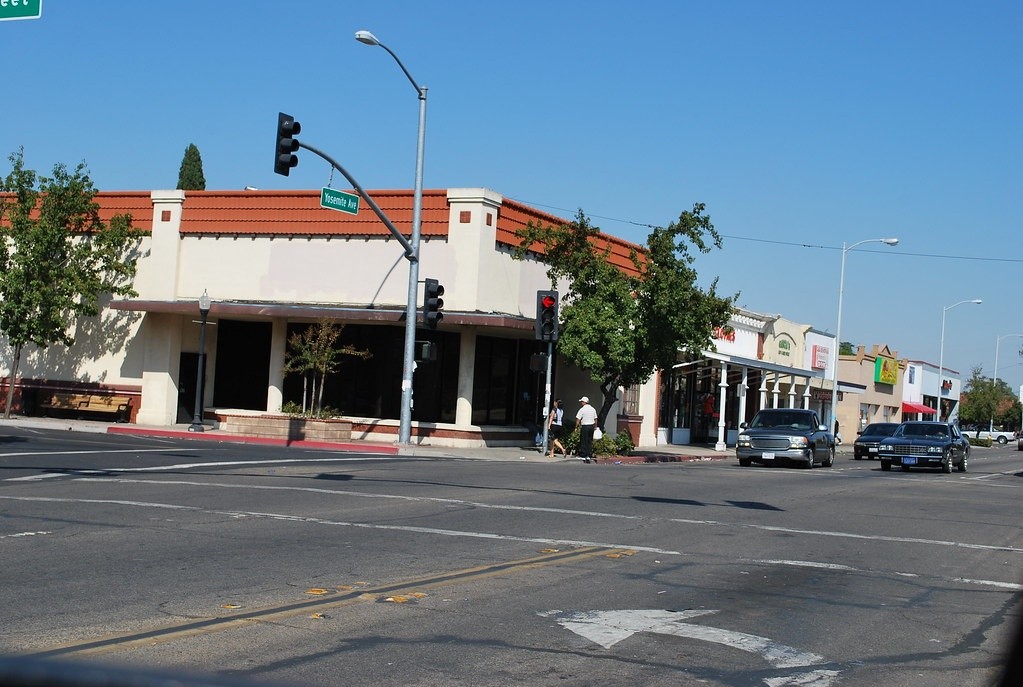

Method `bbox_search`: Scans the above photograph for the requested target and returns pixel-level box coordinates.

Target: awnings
[902,402,937,415]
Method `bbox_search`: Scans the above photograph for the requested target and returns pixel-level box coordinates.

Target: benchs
[41,392,133,423]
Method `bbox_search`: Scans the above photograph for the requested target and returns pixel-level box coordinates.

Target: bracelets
[575,426,577,429]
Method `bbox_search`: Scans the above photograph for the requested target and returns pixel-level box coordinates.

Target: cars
[1016,431,1023,450]
[735,408,837,468]
[877,420,972,473]
[850,422,904,461]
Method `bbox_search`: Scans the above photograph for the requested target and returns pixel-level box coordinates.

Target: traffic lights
[534,290,560,342]
[530,354,549,374]
[423,277,445,326]
[413,340,436,364]
[274,112,299,178]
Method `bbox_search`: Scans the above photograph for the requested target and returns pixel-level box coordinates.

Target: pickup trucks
[961,425,1018,444]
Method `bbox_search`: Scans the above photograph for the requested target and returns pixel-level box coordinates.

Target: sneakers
[586,456,591,461]
[576,455,586,461]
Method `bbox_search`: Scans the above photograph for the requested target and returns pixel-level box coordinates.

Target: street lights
[185,287,211,434]
[988,334,1023,440]
[829,238,900,441]
[354,29,430,448]
[933,298,982,421]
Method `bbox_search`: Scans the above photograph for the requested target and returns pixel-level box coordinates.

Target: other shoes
[547,454,555,459]
[563,449,566,458]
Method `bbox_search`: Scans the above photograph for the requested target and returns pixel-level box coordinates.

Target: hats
[579,396,590,404]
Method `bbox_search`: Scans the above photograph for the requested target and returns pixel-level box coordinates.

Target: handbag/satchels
[593,426,603,440]
[549,407,558,423]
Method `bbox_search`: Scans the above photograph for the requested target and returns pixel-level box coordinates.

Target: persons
[574,397,597,460]
[834,417,843,445]
[546,399,566,459]
